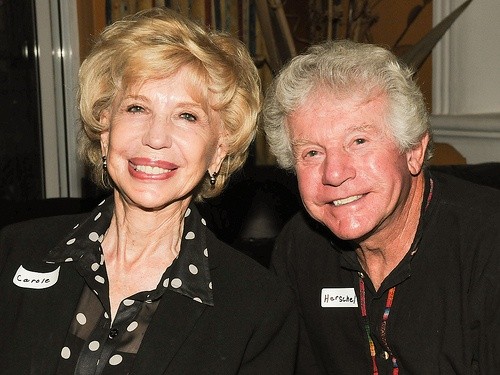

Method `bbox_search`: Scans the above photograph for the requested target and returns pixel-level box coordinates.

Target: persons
[262,39,499,375]
[0,6,301,375]
